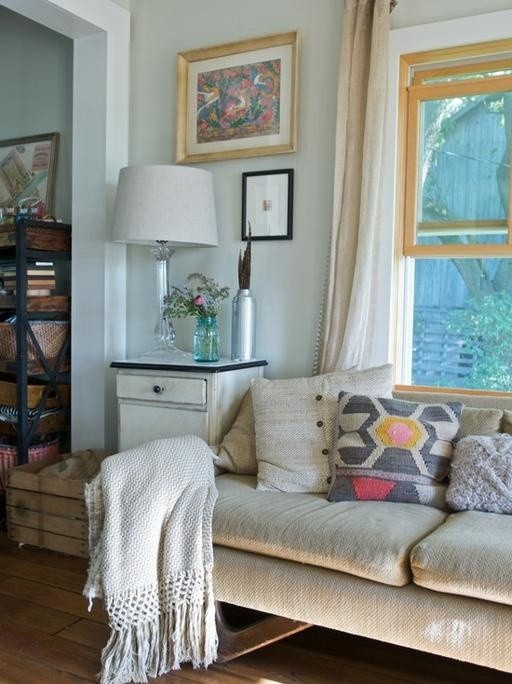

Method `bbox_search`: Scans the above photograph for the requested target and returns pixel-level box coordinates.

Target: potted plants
[230,221,256,362]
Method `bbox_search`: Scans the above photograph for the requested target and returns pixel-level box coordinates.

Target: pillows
[453,407,504,443]
[444,434,512,515]
[328,390,464,514]
[214,365,361,475]
[249,364,393,494]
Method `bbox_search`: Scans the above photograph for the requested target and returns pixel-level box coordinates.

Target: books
[0,255,56,296]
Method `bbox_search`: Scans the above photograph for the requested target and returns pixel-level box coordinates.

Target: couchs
[84,391,512,684]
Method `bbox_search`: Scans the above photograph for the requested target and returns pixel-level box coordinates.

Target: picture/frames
[176,29,301,164]
[0,132,60,218]
[241,168,294,241]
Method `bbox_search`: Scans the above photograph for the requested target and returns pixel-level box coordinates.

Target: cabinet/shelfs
[109,357,268,453]
[0,220,71,493]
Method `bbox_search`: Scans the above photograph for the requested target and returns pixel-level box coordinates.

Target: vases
[193,316,219,361]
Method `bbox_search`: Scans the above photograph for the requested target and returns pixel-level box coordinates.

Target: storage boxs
[1,320,112,560]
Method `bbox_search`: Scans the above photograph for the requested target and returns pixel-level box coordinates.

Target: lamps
[111,164,219,359]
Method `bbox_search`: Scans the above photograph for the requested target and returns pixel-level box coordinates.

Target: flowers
[163,273,230,357]
